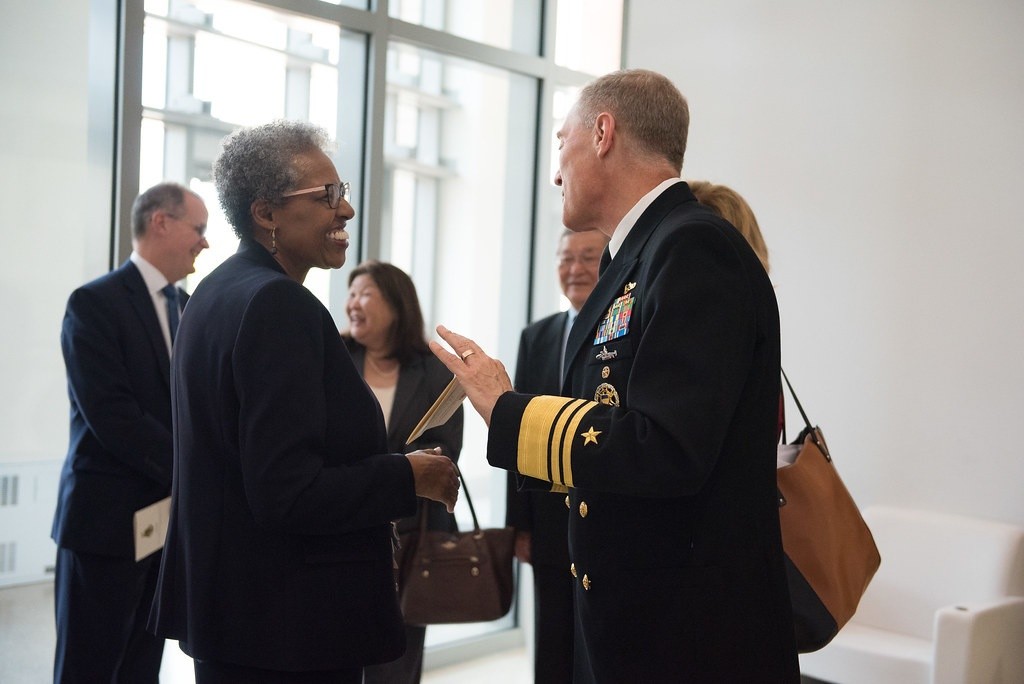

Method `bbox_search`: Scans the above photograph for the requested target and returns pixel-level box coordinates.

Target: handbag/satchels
[392,457,516,624]
[766,422,882,653]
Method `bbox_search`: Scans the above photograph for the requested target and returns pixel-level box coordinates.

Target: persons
[428,70,803,684]
[339,258,465,684]
[145,122,461,684]
[48,182,210,683]
[504,225,611,684]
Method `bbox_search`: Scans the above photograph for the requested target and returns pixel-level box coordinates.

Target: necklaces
[364,350,400,376]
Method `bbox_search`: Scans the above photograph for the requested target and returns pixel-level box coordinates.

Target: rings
[460,348,476,360]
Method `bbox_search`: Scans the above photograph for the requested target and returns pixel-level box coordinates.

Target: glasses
[280,183,352,205]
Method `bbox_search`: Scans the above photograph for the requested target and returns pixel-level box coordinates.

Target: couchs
[794,502,1024,683]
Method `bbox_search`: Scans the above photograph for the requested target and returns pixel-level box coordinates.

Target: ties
[166,284,179,343]
